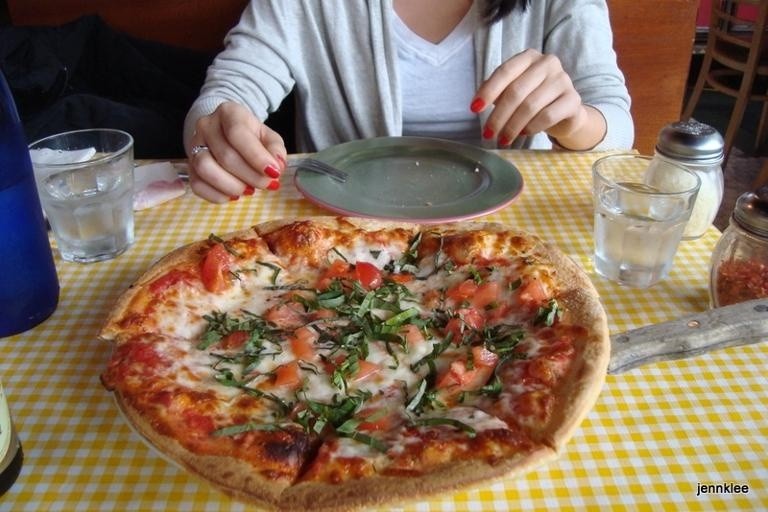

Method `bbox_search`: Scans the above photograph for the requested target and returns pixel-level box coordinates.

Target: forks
[150,158,350,183]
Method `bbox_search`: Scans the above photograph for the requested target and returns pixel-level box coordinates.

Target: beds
[0,151,768,512]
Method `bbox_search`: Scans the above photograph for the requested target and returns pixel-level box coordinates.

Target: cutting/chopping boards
[104,215,763,512]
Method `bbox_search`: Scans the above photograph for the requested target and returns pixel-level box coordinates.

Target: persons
[183,0,634,204]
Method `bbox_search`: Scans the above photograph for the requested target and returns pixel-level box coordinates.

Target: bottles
[707,183,767,310]
[0,68,61,338]
[0,380,27,495]
[646,113,725,240]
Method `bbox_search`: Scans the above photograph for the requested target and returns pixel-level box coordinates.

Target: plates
[294,135,525,222]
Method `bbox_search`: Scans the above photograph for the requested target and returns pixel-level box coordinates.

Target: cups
[590,150,702,290]
[24,130,144,266]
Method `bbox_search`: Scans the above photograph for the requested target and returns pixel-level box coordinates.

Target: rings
[191,145,208,159]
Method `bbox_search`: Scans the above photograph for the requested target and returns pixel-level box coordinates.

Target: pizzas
[97,214,611,512]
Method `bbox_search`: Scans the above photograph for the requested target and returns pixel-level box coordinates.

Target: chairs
[681,0,768,171]
